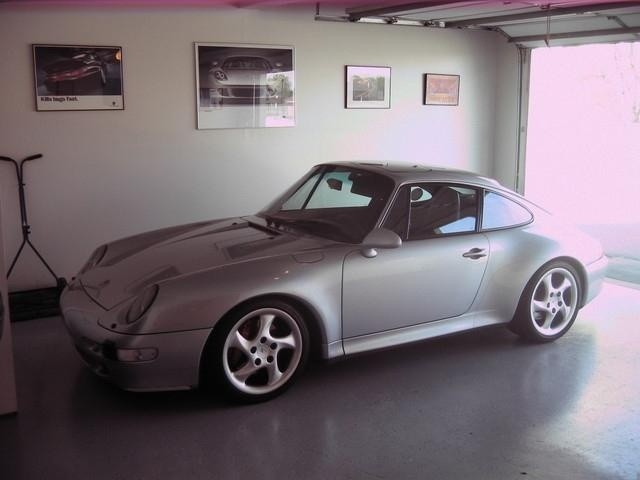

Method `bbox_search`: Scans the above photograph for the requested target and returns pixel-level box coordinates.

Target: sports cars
[60,156,610,405]
[47,51,110,95]
[206,56,287,105]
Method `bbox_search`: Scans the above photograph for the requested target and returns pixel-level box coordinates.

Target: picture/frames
[31,42,124,112]
[192,41,297,130]
[343,64,392,109]
[423,73,461,106]
